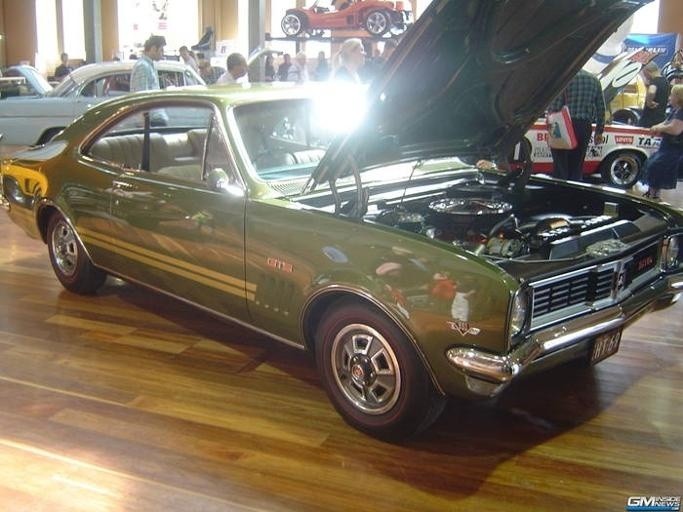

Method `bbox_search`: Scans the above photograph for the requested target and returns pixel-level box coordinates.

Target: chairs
[89,124,324,182]
[191,26,213,57]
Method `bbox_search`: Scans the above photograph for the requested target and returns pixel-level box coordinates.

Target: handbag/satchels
[546,105,578,150]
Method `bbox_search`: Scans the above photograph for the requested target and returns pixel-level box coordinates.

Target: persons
[544,67,607,180]
[665,49,683,116]
[177,36,395,86]
[639,83,683,200]
[54,53,74,83]
[638,61,669,129]
[130,35,167,93]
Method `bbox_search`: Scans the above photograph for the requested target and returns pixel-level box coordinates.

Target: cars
[2,59,214,147]
[499,47,666,189]
[281,0,413,36]
[1,0,683,441]
